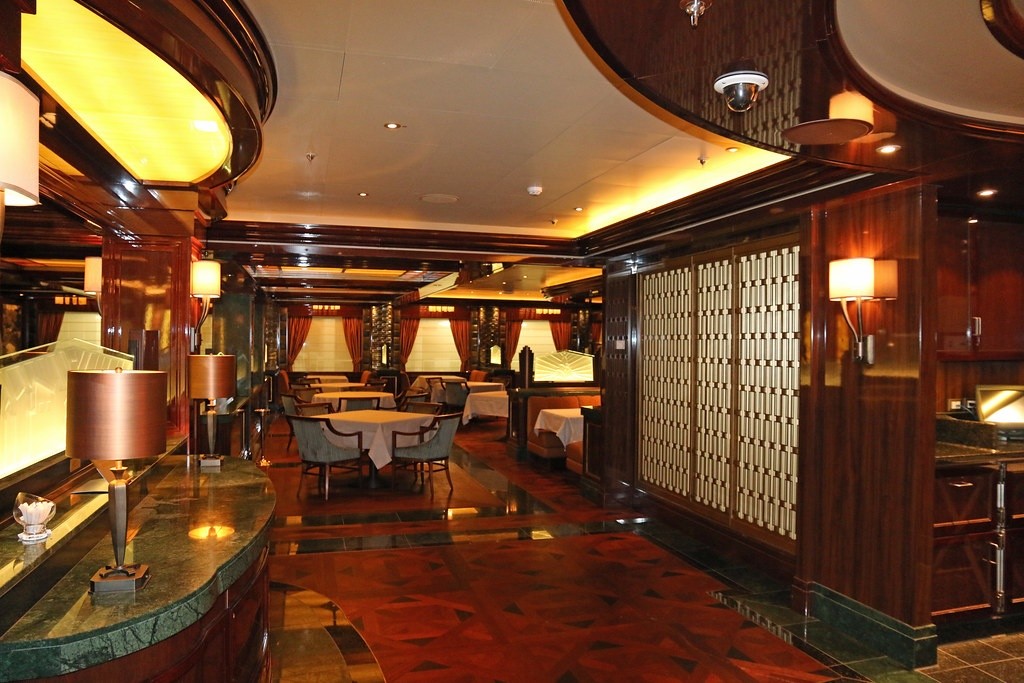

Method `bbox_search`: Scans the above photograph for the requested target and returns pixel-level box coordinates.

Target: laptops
[975,385,1024,441]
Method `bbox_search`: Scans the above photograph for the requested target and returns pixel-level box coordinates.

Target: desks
[0,453,280,683]
[406,375,467,394]
[305,375,349,383]
[312,392,398,411]
[462,391,510,426]
[534,408,593,449]
[433,382,506,393]
[305,382,366,392]
[308,410,440,488]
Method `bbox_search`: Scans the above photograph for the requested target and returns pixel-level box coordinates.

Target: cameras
[713,70,770,112]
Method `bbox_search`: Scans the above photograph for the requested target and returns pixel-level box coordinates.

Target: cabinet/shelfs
[929,468,1024,621]
[937,216,1023,360]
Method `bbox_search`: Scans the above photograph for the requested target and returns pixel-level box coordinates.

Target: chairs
[278,368,513,515]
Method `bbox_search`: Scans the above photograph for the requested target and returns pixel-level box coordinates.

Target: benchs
[566,441,586,475]
[527,394,601,459]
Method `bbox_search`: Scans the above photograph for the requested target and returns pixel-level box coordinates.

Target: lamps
[190,258,222,351]
[871,260,897,351]
[68,365,167,593]
[829,90,875,130]
[187,476,236,542]
[85,255,103,315]
[185,351,240,467]
[830,258,877,368]
[0,69,40,242]
